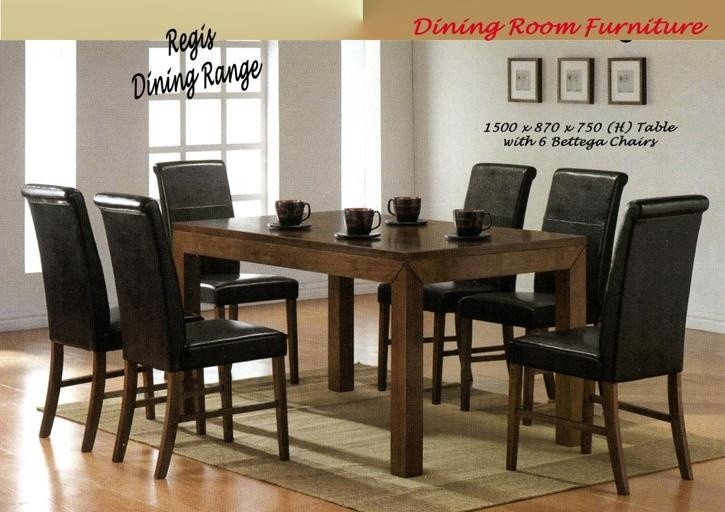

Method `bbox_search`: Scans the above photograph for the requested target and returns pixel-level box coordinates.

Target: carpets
[36,361,725,511]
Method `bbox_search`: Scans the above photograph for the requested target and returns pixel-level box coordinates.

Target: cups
[265,196,494,242]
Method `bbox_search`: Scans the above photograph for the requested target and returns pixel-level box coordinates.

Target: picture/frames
[608,55,646,107]
[508,57,543,103]
[556,56,596,106]
